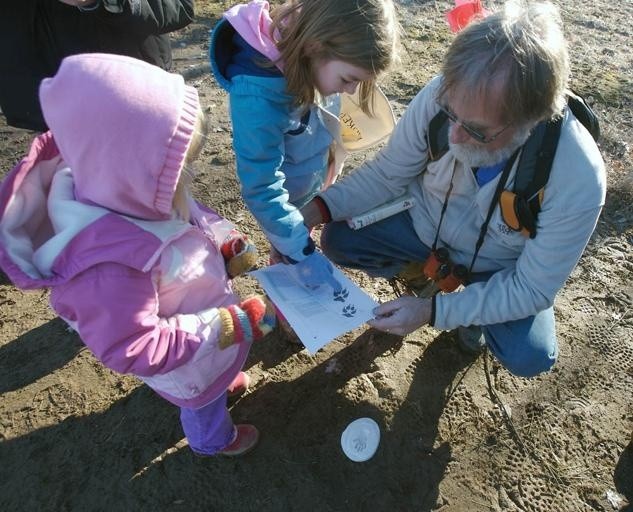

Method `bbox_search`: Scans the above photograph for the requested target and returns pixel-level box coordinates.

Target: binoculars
[422,248,467,293]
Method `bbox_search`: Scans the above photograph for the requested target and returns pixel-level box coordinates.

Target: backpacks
[427,89,602,239]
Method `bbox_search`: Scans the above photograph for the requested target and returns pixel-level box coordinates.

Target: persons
[209,0,402,345]
[269,2,607,377]
[0,53,276,456]
[0,0,195,133]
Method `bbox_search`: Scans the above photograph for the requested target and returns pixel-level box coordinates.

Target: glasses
[435,98,516,143]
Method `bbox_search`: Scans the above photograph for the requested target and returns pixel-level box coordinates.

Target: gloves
[283,240,342,293]
[220,229,258,278]
[218,294,278,352]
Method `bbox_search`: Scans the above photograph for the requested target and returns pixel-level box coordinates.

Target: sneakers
[397,262,443,300]
[212,424,259,458]
[457,325,487,357]
[224,371,250,398]
